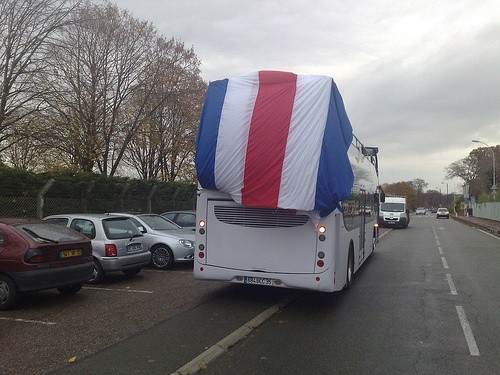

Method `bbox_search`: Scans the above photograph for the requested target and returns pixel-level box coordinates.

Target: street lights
[442,182,448,209]
[472,140,496,200]
[445,176,456,214]
[435,187,442,207]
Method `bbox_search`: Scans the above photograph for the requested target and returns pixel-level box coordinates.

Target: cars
[436,208,450,219]
[415,207,426,215]
[74,213,196,269]
[0,216,95,311]
[155,209,196,232]
[425,207,437,213]
[41,212,152,285]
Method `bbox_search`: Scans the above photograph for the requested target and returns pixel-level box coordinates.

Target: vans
[379,195,409,229]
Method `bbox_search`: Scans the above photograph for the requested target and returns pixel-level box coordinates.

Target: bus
[193,70,386,295]
